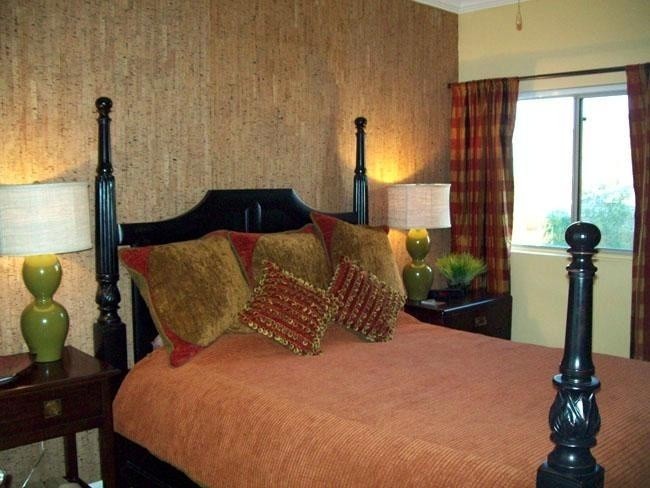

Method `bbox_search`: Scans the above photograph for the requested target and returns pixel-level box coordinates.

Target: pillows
[238,260,344,356]
[309,210,408,298]
[118,230,257,367]
[228,223,334,292]
[327,256,406,343]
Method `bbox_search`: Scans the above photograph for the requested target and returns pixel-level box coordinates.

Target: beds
[89,91,649,488]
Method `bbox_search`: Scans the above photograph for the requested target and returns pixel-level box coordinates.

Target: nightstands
[0,345,124,488]
[404,286,513,341]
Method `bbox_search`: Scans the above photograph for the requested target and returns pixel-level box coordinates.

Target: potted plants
[433,248,488,298]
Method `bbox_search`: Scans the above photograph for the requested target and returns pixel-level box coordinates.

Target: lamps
[386,178,456,302]
[0,181,95,368]
